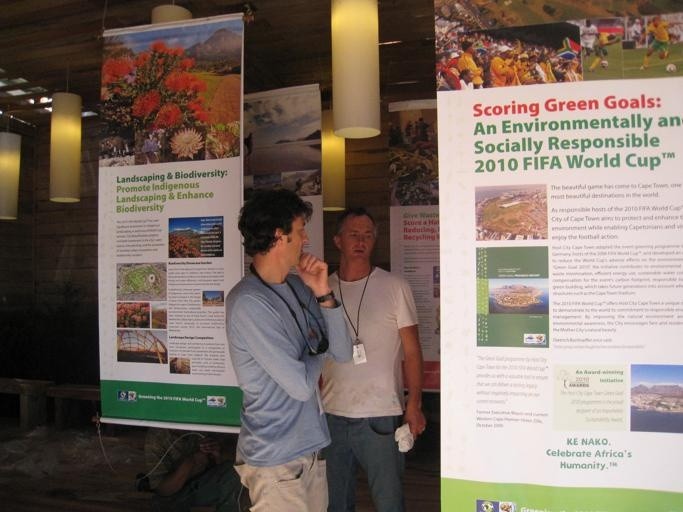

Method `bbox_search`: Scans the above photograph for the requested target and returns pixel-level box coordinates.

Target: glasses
[292,301,329,357]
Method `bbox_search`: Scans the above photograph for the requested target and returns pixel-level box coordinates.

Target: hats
[461,40,473,49]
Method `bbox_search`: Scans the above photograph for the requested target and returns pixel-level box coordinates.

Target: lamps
[320,1,381,211]
[0,105,21,220]
[47,69,81,203]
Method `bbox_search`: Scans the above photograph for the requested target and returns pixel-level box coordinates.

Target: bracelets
[315,291,335,304]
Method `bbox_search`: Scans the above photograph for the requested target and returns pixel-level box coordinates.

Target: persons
[436,15,674,94]
[313,207,426,510]
[222,188,356,511]
